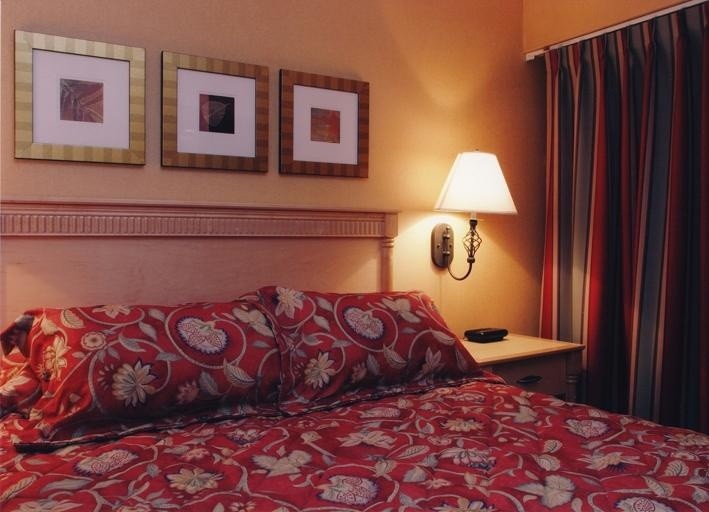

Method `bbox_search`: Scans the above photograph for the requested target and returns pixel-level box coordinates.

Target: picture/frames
[160,51,269,173]
[279,69,368,178]
[14,29,145,166]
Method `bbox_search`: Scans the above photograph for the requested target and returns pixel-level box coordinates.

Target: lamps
[431,150,518,282]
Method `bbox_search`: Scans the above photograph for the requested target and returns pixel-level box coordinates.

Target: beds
[0,379,709,511]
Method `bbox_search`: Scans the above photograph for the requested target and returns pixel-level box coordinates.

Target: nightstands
[460,332,585,403]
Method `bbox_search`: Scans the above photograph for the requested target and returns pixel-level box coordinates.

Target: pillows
[234,285,506,416]
[0,299,285,448]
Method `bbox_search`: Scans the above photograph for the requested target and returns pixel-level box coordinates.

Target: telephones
[464,327,508,343]
